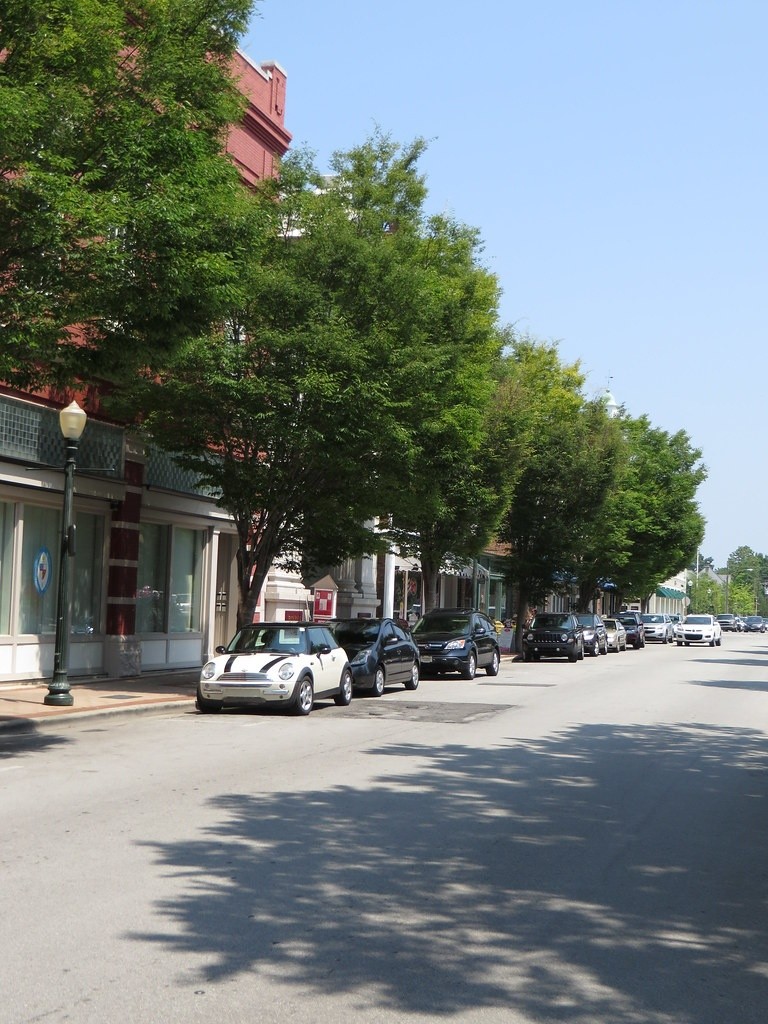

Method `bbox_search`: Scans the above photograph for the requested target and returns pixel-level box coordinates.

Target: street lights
[44,401,88,707]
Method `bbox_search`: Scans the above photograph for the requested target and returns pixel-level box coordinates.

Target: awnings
[655,587,687,600]
[393,537,490,581]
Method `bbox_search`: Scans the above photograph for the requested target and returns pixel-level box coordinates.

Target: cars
[668,615,683,637]
[574,613,674,657]
[674,614,722,646]
[734,616,768,633]
[196,621,357,714]
[322,617,422,697]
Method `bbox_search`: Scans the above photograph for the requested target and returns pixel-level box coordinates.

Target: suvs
[408,607,501,679]
[522,612,584,662]
[716,614,737,632]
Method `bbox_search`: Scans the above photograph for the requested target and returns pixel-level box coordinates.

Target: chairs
[256,631,276,649]
